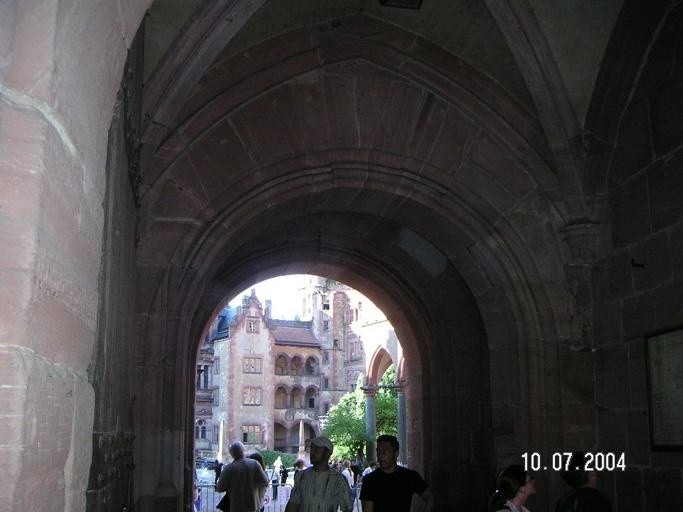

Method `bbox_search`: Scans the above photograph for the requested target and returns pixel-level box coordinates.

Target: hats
[310,437,333,453]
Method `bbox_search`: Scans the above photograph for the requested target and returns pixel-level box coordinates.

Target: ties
[270,471,274,480]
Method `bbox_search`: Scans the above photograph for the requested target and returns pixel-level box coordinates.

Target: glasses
[524,476,536,487]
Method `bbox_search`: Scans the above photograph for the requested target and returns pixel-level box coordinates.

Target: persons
[552,451,614,512]
[485,463,538,512]
[192,434,376,512]
[358,434,436,511]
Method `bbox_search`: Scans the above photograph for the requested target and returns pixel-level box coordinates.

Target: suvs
[204,458,217,470]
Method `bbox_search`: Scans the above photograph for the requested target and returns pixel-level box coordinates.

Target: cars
[264,465,294,481]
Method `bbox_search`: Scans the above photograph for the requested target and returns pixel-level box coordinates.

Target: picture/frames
[644,325,683,453]
